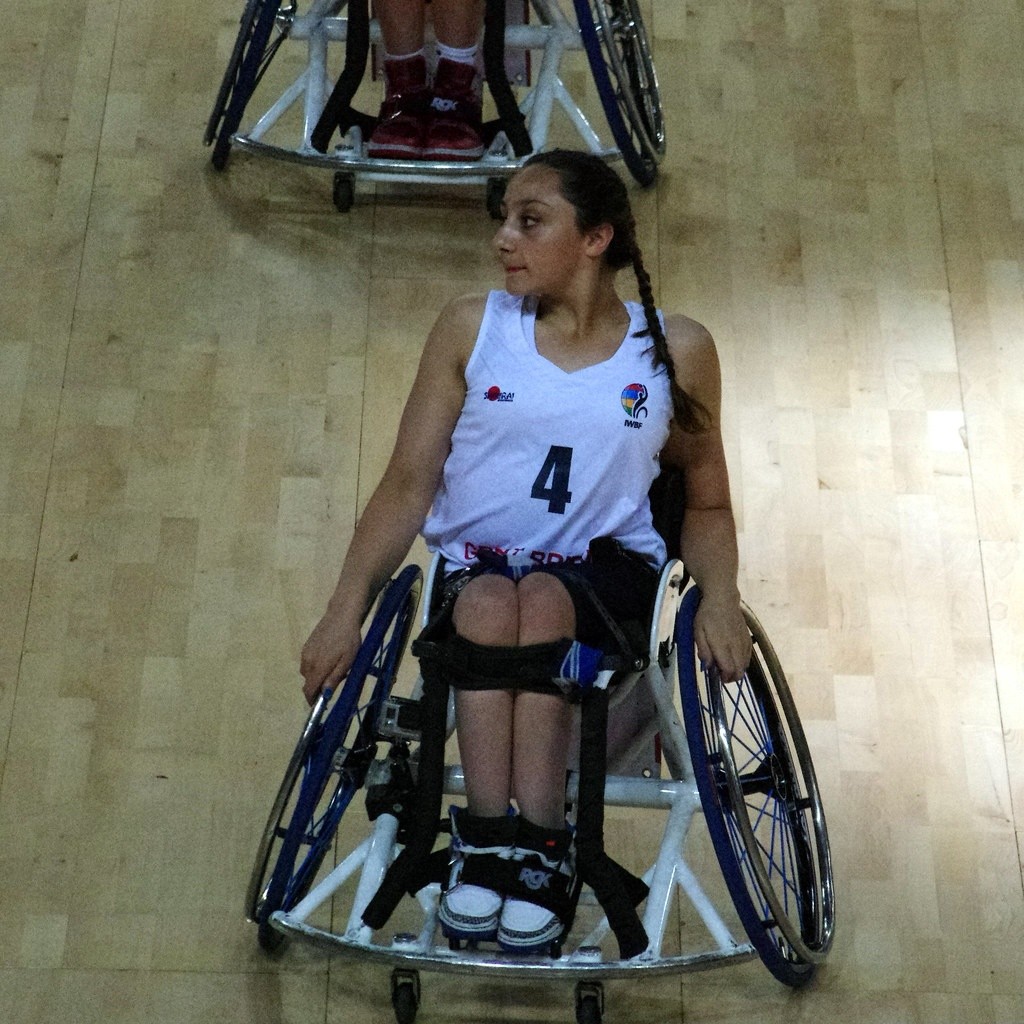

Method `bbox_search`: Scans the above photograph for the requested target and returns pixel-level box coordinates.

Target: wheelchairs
[245,464,835,1024]
[197,2,669,214]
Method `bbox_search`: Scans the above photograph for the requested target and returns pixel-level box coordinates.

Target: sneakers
[437,811,503,941]
[498,830,578,950]
[367,60,429,159]
[422,57,485,158]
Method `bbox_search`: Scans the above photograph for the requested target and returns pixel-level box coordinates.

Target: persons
[366,0,487,162]
[299,147,753,955]
